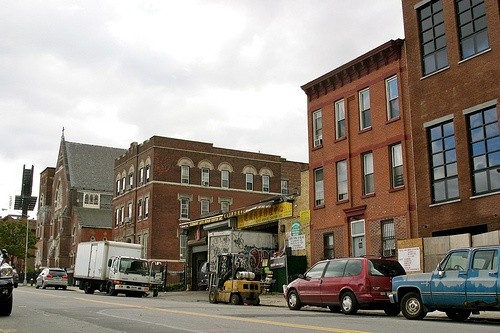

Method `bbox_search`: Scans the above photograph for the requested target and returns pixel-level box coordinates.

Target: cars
[283,255,408,314]
[0,248,15,317]
[12,266,19,288]
[35,268,68,290]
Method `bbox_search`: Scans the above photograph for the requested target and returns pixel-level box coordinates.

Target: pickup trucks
[389,246,500,321]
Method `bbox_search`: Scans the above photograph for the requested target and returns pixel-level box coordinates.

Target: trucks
[74,241,164,297]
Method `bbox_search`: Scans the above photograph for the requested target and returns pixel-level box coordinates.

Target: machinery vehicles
[208,252,262,305]
